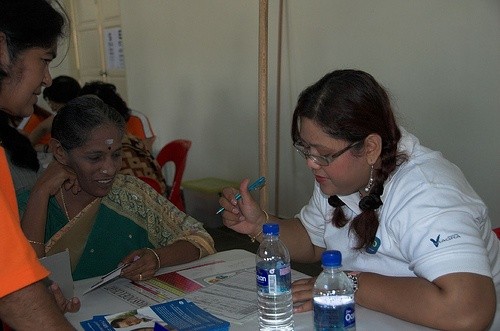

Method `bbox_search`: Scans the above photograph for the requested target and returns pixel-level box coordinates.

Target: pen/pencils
[216,176,265,215]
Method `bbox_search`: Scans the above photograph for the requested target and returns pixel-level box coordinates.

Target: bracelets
[249,208,269,239]
[27,240,43,246]
[147,247,160,268]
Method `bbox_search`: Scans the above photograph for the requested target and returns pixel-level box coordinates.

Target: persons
[22,93,215,284]
[15,75,169,194]
[216,69,500,331]
[0,0,81,331]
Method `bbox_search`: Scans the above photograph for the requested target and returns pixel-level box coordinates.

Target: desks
[65,248,440,331]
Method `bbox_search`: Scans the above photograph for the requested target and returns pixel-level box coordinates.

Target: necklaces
[60,185,70,223]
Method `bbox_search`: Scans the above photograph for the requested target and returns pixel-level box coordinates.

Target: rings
[140,274,143,281]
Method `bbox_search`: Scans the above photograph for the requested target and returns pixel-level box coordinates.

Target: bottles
[313,250,357,331]
[255,223,295,331]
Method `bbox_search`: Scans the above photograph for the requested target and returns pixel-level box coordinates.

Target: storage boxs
[181,177,240,229]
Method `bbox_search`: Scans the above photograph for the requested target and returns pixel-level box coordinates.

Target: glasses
[291,139,359,166]
